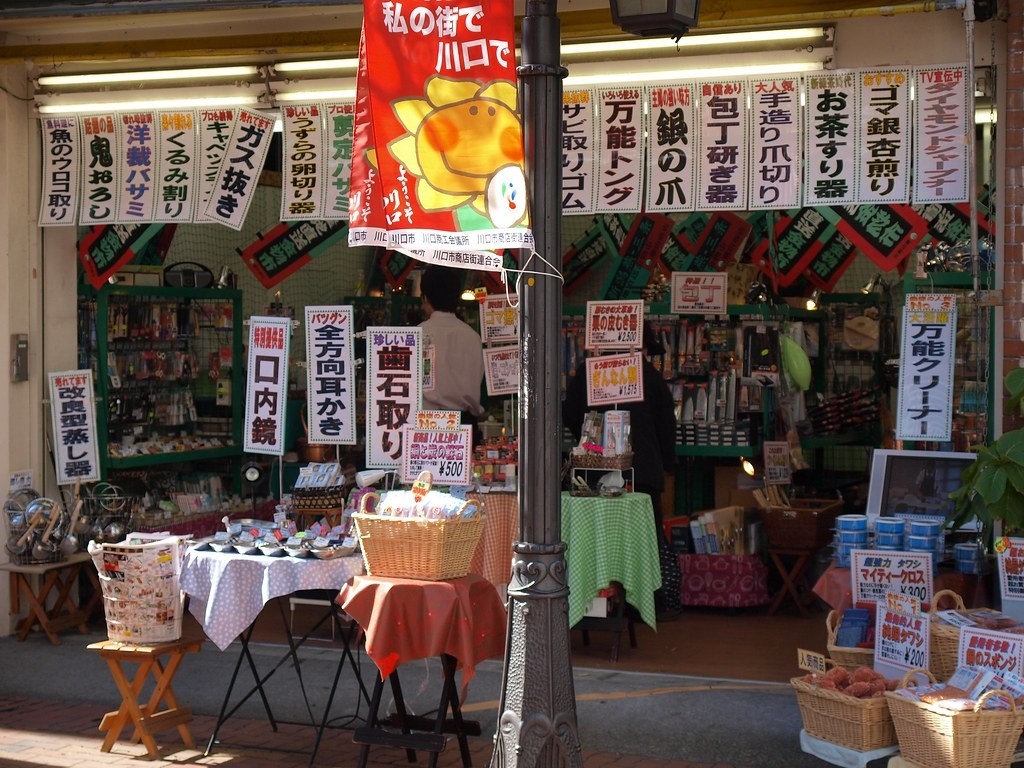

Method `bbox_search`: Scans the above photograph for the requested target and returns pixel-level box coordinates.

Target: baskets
[290,485,345,509]
[790,659,898,753]
[929,590,1023,681]
[350,492,488,579]
[826,608,876,670]
[884,669,1024,768]
[577,452,634,470]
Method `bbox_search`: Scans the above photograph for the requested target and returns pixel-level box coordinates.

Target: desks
[179,535,381,768]
[559,491,662,665]
[332,573,507,768]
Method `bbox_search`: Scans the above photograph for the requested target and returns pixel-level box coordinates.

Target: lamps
[609,0,701,52]
[975,94,997,124]
[267,47,522,78]
[559,27,836,53]
[32,83,273,117]
[561,47,835,86]
[269,79,356,105]
[32,62,267,88]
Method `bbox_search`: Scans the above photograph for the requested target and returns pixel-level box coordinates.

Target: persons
[408,266,487,451]
[563,321,683,620]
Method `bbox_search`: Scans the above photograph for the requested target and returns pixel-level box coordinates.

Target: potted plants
[939,366,1023,611]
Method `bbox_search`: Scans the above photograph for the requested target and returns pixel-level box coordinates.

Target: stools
[759,545,822,617]
[0,552,93,647]
[87,635,207,760]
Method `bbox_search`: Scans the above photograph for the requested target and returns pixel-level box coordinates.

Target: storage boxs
[572,467,634,493]
[759,497,844,552]
[676,553,770,610]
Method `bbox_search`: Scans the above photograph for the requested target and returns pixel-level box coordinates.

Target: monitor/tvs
[867,449,983,533]
